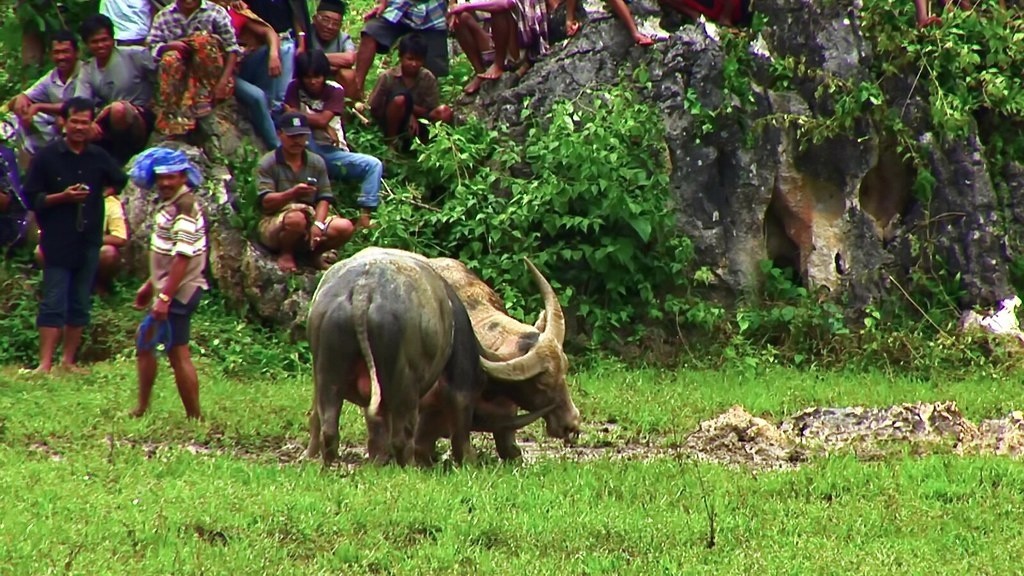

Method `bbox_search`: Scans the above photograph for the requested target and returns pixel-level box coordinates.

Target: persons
[123,148,213,426]
[279,49,383,235]
[0,144,27,258]
[32,189,132,300]
[252,114,354,276]
[913,0,975,29]
[25,96,130,381]
[0,0,752,152]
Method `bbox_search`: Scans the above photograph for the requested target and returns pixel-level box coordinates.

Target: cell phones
[78,184,90,192]
[307,176,317,186]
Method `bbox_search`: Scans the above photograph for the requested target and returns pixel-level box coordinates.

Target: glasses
[317,13,343,30]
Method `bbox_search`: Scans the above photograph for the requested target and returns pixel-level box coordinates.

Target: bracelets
[157,293,173,307]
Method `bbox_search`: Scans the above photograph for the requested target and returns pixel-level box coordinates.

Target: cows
[408,251,585,467]
[296,243,490,478]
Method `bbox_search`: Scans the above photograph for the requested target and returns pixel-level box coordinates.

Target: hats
[317,0,345,17]
[276,111,312,136]
